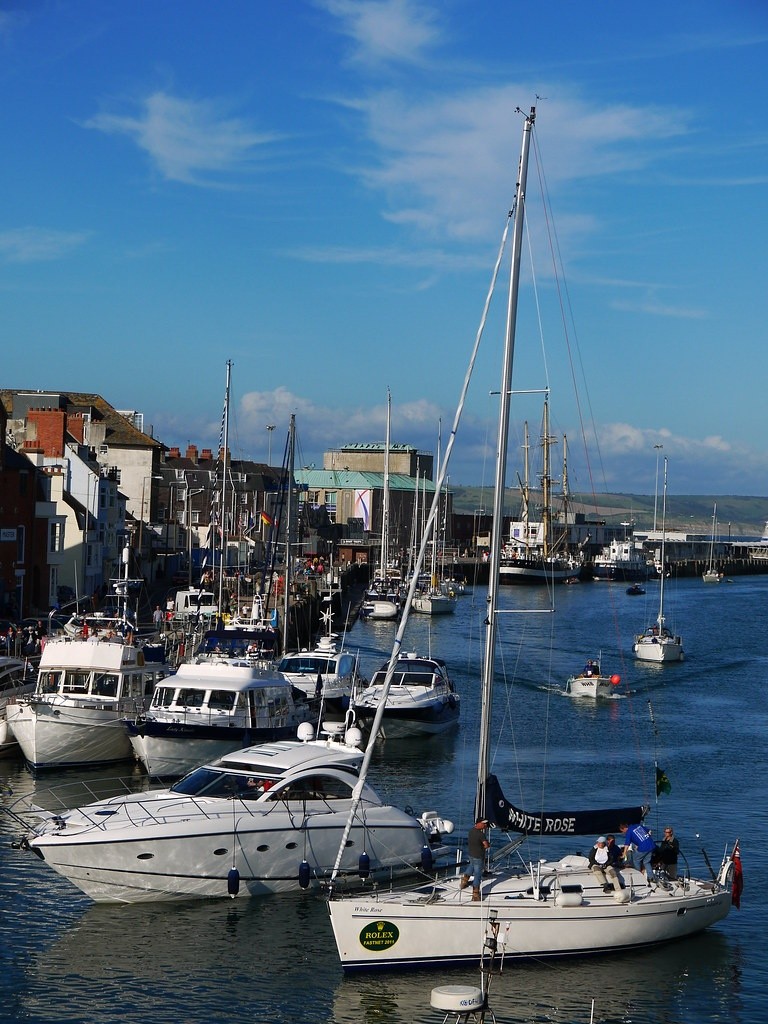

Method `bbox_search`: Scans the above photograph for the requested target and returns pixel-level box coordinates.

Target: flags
[275,517,277,527]
[731,844,744,911]
[656,767,672,801]
[262,511,272,528]
[238,512,242,530]
[218,525,222,538]
[250,513,254,533]
[315,670,324,698]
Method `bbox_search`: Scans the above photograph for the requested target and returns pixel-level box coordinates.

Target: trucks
[227,541,249,564]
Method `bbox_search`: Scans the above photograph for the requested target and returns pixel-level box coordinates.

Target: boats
[567,671,619,700]
[592,536,656,580]
[626,584,646,596]
[4,616,174,769]
[562,578,580,586]
[653,560,670,579]
[4,720,457,903]
[278,637,368,708]
[119,654,321,782]
[354,650,460,740]
[0,657,38,753]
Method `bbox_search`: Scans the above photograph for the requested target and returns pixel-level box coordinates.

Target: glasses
[18,630,22,631]
[666,832,671,834]
[249,780,254,782]
[589,663,591,664]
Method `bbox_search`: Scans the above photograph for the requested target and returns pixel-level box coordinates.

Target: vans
[175,589,218,625]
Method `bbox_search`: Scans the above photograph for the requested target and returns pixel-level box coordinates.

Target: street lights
[139,475,162,569]
[265,492,279,535]
[83,467,108,608]
[265,424,275,468]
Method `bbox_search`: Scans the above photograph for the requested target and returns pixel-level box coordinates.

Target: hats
[592,662,597,665]
[27,626,33,630]
[476,817,488,824]
[606,835,616,840]
[597,836,606,843]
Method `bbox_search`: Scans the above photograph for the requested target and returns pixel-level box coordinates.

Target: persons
[588,834,621,893]
[620,823,657,883]
[659,826,679,881]
[653,624,659,635]
[461,817,490,901]
[0,554,325,659]
[582,659,599,678]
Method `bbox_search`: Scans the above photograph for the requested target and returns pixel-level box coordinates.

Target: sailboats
[361,385,474,621]
[192,360,294,663]
[634,456,685,662]
[701,500,724,583]
[497,386,590,585]
[323,99,738,969]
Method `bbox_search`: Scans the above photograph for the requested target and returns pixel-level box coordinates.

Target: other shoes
[460,876,469,889]
[602,884,610,892]
[472,895,485,901]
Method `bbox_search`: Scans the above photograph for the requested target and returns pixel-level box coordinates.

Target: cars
[23,617,64,633]
[53,614,79,625]
[99,606,138,624]
[0,620,22,637]
